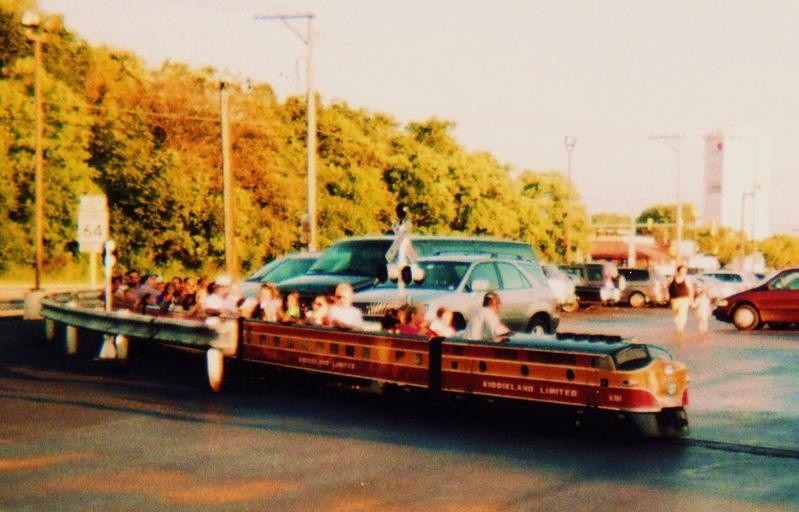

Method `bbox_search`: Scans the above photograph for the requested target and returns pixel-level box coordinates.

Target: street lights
[563,134,577,245]
[19,10,58,292]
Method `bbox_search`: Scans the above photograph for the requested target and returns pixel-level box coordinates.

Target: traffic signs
[78,197,109,254]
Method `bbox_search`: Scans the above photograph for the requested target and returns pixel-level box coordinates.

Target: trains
[39,276,695,454]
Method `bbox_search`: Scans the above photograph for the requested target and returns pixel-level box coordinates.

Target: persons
[384,292,511,343]
[111,268,363,330]
[663,266,694,331]
[691,288,711,333]
[603,257,620,288]
